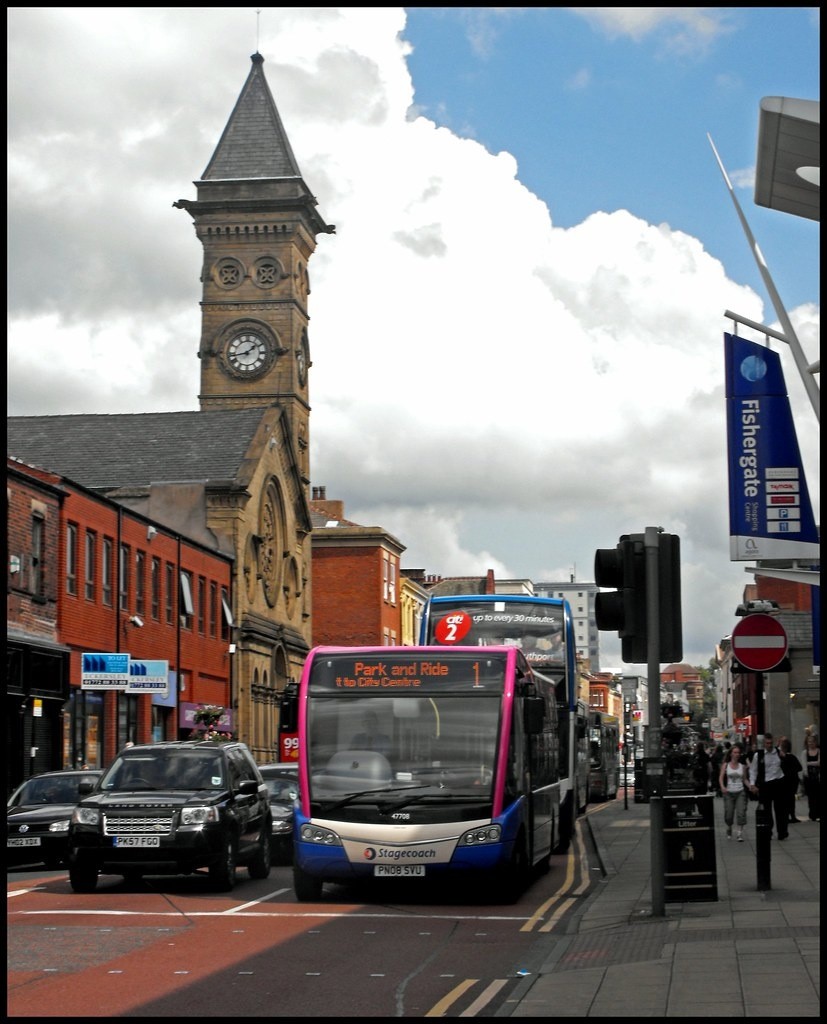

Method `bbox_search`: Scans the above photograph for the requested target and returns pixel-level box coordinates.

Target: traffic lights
[592,536,652,665]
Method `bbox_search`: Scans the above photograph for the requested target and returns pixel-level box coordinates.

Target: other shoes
[815,817,821,822]
[778,831,789,840]
[727,829,732,840]
[737,835,744,842]
[788,818,801,824]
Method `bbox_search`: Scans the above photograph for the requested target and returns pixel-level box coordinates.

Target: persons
[663,723,820,842]
[349,710,391,757]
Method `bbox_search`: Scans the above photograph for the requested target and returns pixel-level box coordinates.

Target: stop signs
[732,612,788,670]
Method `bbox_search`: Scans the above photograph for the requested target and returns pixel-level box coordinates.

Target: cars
[7,770,103,872]
[249,762,299,865]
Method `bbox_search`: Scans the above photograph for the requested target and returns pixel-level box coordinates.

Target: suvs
[66,741,273,891]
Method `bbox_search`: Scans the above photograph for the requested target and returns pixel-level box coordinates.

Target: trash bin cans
[660,795,720,904]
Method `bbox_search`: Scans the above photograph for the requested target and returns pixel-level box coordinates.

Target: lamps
[222,644,238,659]
[122,614,144,635]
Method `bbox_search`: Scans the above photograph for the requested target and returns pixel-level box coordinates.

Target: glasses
[732,752,740,754]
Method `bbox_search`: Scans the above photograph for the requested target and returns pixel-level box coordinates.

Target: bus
[292,646,566,906]
[413,593,624,839]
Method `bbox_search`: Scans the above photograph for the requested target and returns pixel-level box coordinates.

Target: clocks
[295,325,313,387]
[206,317,289,383]
